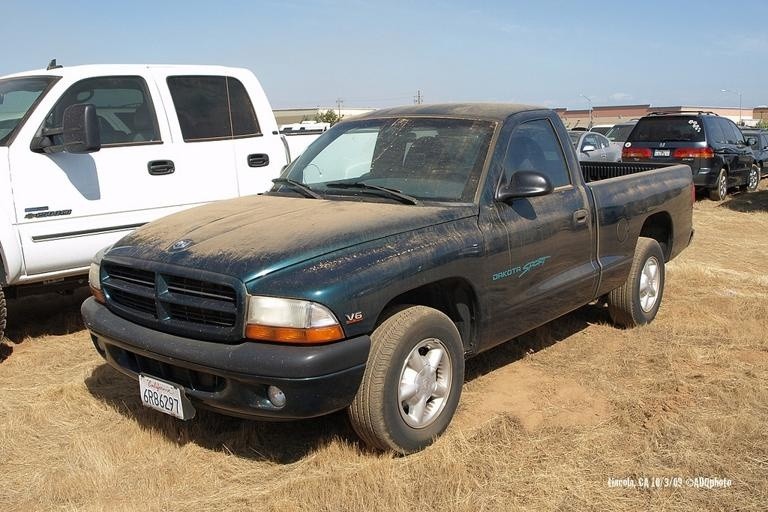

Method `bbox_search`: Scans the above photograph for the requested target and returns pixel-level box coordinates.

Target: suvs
[622,110,760,202]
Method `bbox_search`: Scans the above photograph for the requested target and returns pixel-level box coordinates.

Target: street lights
[721,89,742,129]
[579,93,592,129]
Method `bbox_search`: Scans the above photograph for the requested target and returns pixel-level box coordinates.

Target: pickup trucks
[80,103,696,456]
[0,58,439,351]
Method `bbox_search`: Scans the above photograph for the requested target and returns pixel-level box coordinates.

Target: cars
[564,118,767,177]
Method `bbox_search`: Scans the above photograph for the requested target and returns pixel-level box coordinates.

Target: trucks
[277,119,332,134]
